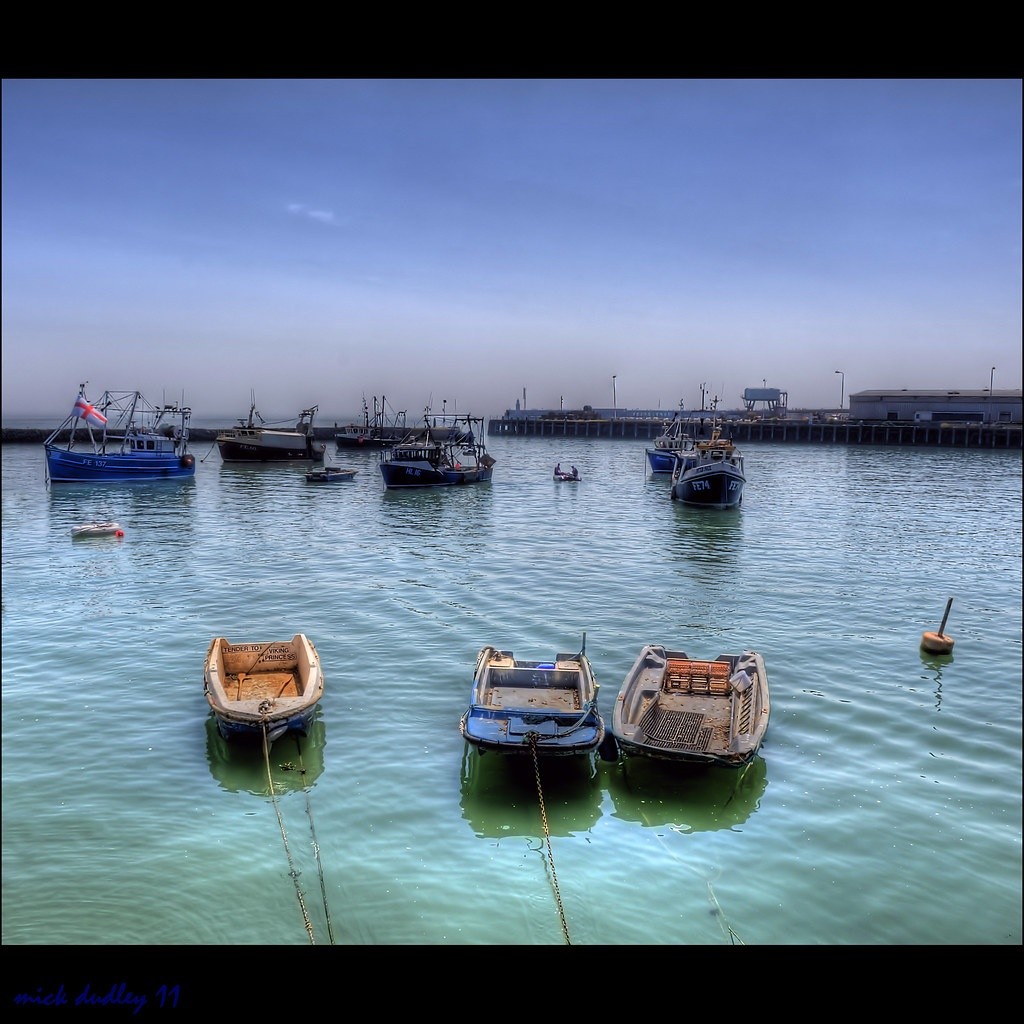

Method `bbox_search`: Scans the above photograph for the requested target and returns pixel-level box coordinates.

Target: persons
[571,466,578,476]
[555,463,565,475]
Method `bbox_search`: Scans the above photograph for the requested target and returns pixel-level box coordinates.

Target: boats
[609,641,771,768]
[552,473,581,482]
[670,394,748,509]
[71,519,118,539]
[333,393,407,447]
[377,411,497,489]
[302,465,359,482]
[216,388,326,462]
[459,630,607,756]
[203,632,325,745]
[643,382,737,473]
[40,381,196,482]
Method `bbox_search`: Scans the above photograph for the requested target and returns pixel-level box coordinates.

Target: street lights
[834,370,844,410]
[612,375,617,419]
[988,367,996,427]
[762,379,767,420]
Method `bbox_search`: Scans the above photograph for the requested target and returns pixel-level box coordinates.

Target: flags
[71,397,108,428]
